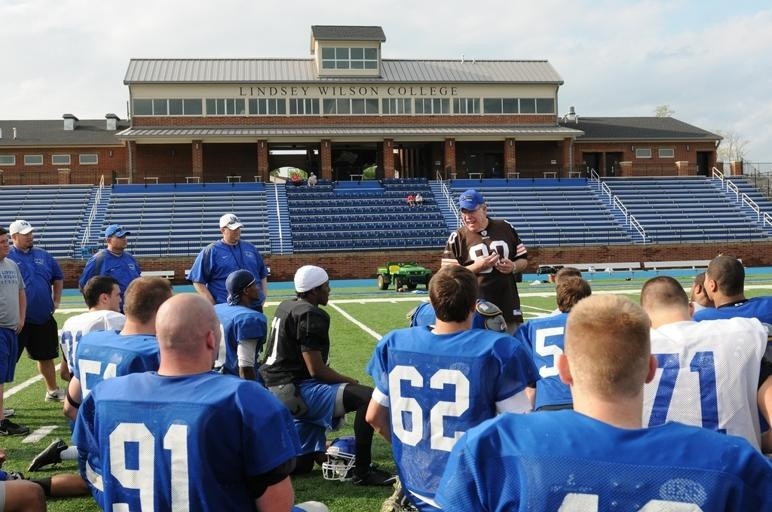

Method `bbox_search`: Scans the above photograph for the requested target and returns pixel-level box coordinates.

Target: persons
[258,264,397,487]
[365,266,541,512]
[210,269,268,387]
[639,275,769,452]
[512,276,592,410]
[78,224,141,315]
[10,275,174,498]
[0,448,47,511]
[72,292,331,511]
[693,256,772,325]
[433,294,772,512]
[688,273,715,318]
[406,263,510,333]
[7,219,66,404]
[0,226,30,437]
[547,267,582,317]
[27,275,127,473]
[187,213,268,315]
[439,189,528,336]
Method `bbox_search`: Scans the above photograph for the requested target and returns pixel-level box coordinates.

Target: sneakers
[3,408,14,417]
[353,468,396,487]
[0,418,29,436]
[27,438,67,472]
[44,390,65,403]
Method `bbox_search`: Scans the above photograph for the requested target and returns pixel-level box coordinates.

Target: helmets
[321,435,357,481]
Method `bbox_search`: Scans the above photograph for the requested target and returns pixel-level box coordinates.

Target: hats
[105,224,131,239]
[458,190,484,211]
[219,213,244,231]
[9,219,34,235]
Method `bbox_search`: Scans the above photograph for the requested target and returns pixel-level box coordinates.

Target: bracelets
[511,261,516,275]
